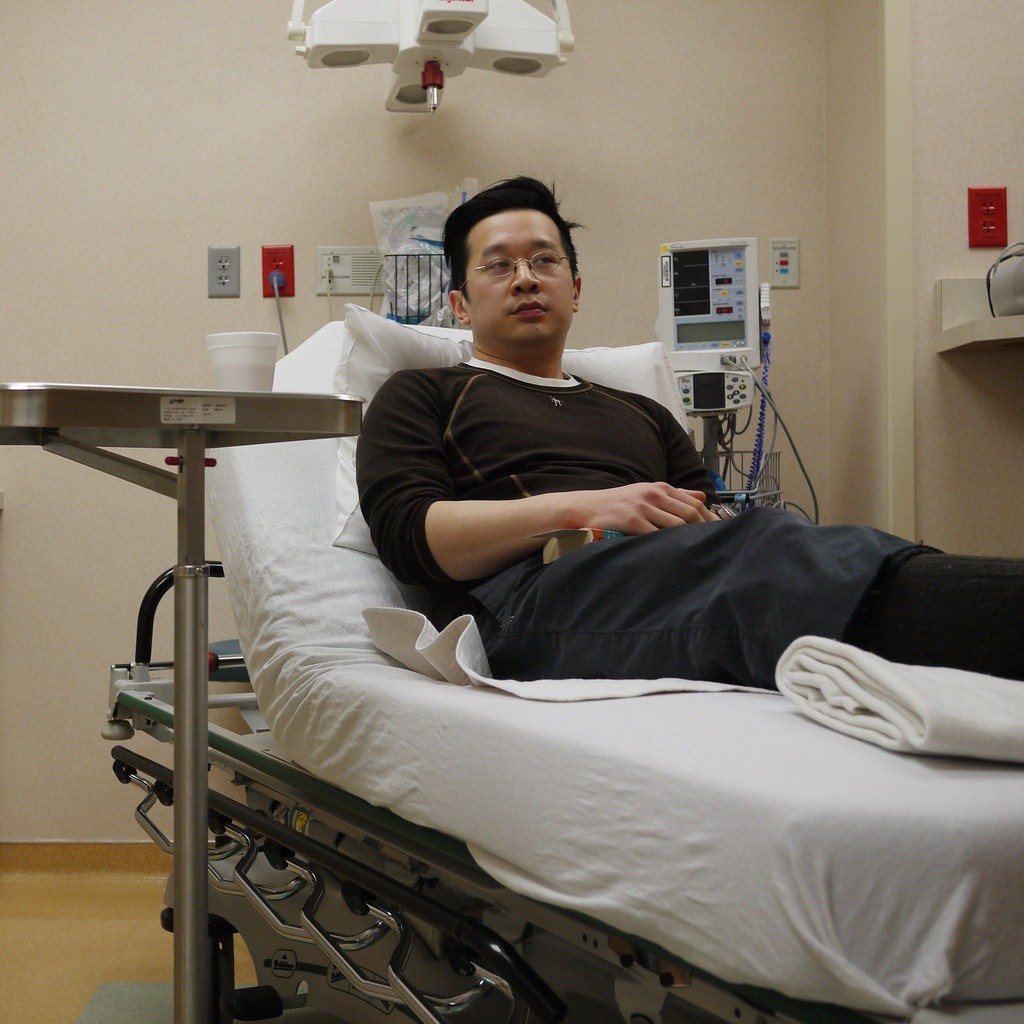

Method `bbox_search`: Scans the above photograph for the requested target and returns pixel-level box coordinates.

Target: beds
[98,323,1024,1024]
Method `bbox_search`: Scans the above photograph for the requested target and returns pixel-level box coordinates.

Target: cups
[205,332,280,392]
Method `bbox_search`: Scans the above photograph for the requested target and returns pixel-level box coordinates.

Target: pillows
[330,303,696,557]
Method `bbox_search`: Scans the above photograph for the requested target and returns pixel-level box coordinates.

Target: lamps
[283,0,575,114]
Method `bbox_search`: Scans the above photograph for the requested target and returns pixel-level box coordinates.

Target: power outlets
[208,245,240,298]
[966,186,1007,250]
[261,244,295,298]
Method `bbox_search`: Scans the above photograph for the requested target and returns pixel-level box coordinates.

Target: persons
[357,176,1024,691]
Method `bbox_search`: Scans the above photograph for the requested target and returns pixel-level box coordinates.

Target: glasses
[459,250,573,291]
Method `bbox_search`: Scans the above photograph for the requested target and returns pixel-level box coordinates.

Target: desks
[1,383,368,1024]
